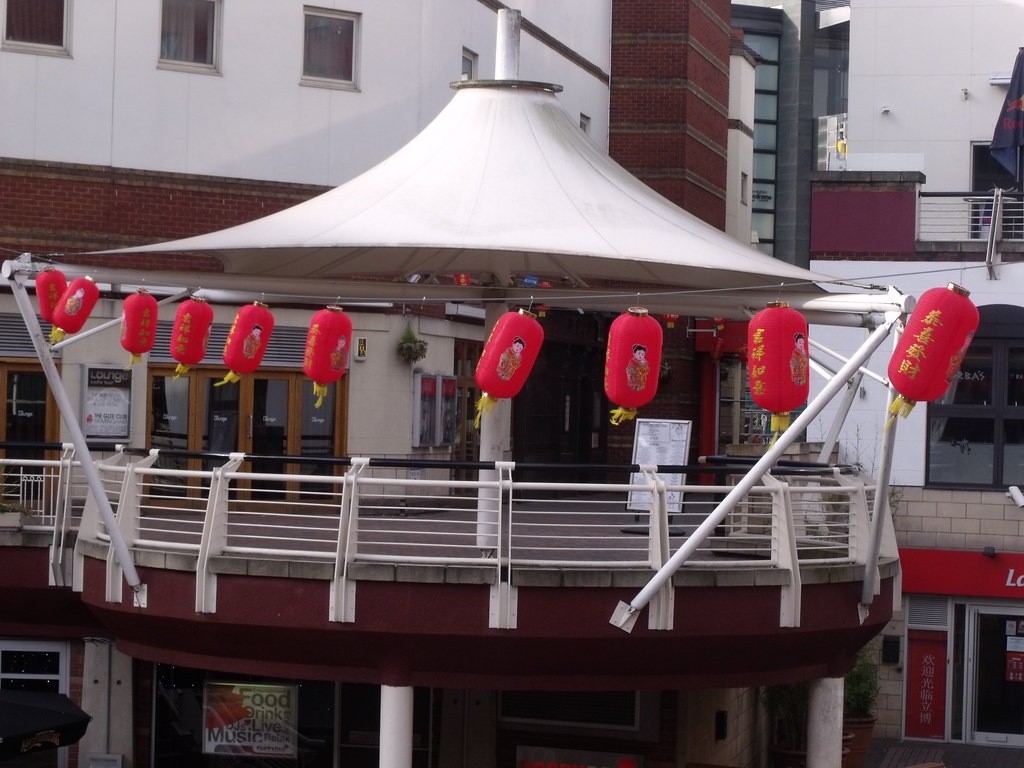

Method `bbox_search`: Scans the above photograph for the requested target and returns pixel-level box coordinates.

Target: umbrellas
[989,48,1024,195]
[0,689,91,759]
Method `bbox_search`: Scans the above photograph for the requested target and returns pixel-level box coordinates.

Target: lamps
[982,546,997,558]
[961,89,971,100]
[1005,485,1024,507]
[988,71,1012,85]
[881,105,889,116]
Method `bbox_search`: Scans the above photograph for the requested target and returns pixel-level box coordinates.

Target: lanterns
[48,275,100,346]
[473,307,544,429]
[119,288,158,371]
[883,282,979,432]
[453,274,471,286]
[604,307,663,425]
[213,300,274,387]
[35,267,67,334]
[304,305,352,408]
[665,313,679,328]
[534,281,551,317]
[169,295,214,381]
[748,300,809,450]
[712,317,725,330]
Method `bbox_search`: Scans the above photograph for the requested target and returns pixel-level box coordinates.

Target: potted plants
[760,658,883,768]
[397,322,427,364]
